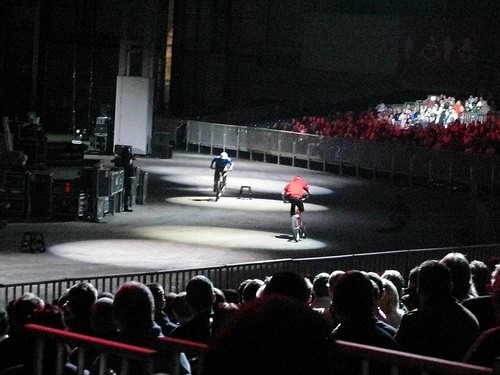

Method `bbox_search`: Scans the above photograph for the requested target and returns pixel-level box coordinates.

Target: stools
[238,186,253,199]
[21,231,46,253]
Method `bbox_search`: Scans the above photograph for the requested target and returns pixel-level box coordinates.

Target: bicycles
[282,194,312,242]
[209,166,233,202]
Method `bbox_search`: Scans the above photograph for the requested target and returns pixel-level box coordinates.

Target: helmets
[221,152,227,159]
[294,176,302,181]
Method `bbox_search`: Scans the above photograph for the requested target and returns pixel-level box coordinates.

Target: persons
[1,252,500,375]
[211,152,234,194]
[212,92,499,174]
[111,147,134,212]
[282,175,312,221]
